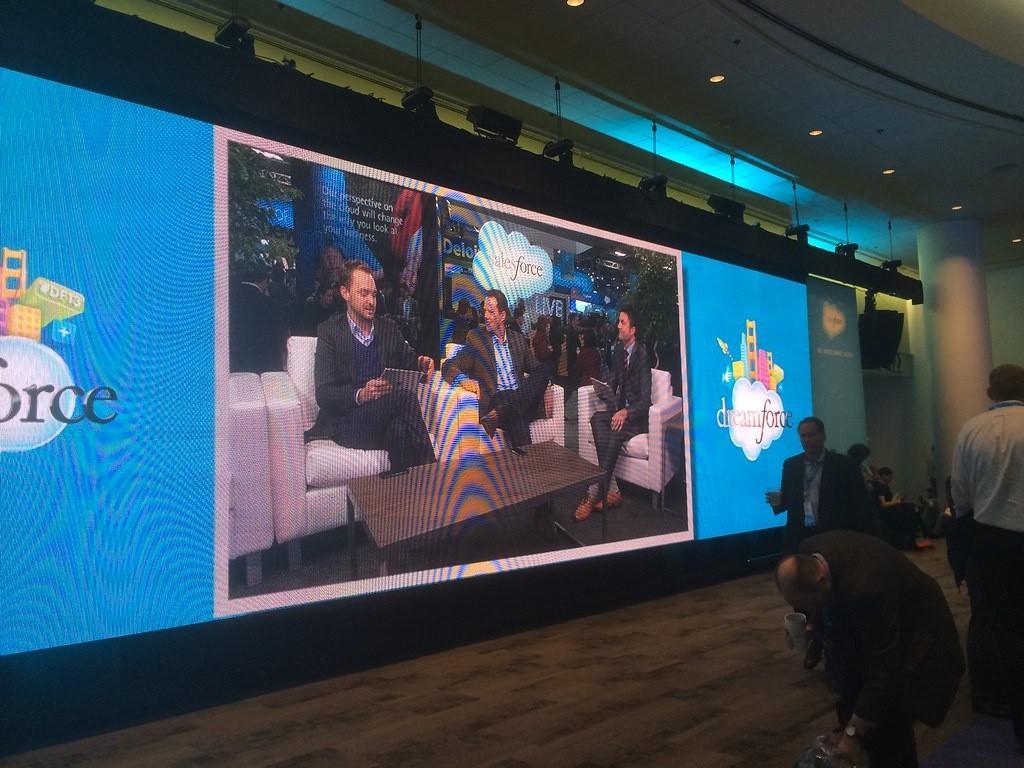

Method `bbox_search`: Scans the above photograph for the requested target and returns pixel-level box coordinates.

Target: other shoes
[803,638,822,669]
[908,542,922,552]
[971,694,1012,717]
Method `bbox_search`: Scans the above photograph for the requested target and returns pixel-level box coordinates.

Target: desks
[661,410,685,514]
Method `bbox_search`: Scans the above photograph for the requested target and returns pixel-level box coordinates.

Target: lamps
[543,139,575,164]
[639,175,668,196]
[400,87,437,121]
[708,194,747,222]
[836,242,859,258]
[215,15,254,54]
[785,224,808,245]
[466,105,524,145]
[882,259,903,272]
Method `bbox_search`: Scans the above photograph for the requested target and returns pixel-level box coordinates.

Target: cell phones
[379,469,408,479]
[511,448,528,457]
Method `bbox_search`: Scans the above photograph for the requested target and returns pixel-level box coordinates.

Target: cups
[768,492,781,505]
[784,613,807,648]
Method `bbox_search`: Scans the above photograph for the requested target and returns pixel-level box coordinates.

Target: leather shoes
[593,491,623,511]
[575,496,597,521]
[481,410,499,439]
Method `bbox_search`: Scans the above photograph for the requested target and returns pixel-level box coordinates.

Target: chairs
[261,334,459,576]
[440,343,564,458]
[230,370,274,588]
[578,367,682,513]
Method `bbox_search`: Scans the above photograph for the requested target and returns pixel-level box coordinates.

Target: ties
[623,348,629,371]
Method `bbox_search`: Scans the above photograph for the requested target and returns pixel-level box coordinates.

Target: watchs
[843,722,864,740]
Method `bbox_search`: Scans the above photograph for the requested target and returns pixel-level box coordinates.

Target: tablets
[379,368,424,391]
[589,377,614,401]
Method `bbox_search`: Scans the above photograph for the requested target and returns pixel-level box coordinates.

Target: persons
[950,364,1024,716]
[846,443,938,552]
[776,531,965,768]
[766,417,861,671]
[229,239,652,522]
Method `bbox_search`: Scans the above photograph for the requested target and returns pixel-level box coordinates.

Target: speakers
[858,310,904,370]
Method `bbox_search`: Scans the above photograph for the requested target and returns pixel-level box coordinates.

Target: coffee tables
[344,440,608,582]
